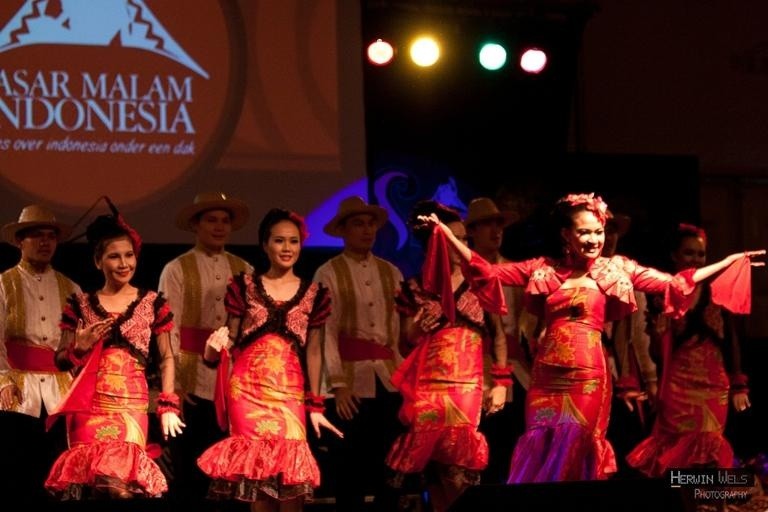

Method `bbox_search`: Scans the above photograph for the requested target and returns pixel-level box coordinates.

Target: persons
[159,187,257,510]
[380,187,768,512]
[0,205,85,512]
[41,213,190,511]
[195,206,348,511]
[307,194,410,511]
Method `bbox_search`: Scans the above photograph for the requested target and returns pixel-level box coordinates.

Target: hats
[176,190,250,232]
[0,205,71,248]
[464,198,519,229]
[605,214,630,236]
[324,195,389,237]
